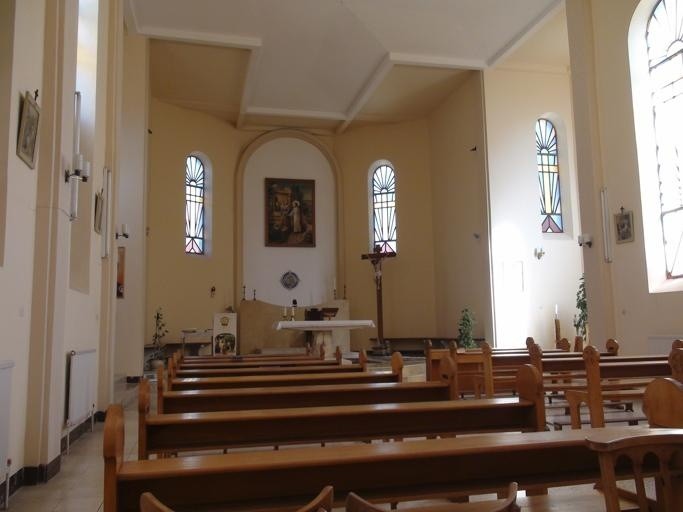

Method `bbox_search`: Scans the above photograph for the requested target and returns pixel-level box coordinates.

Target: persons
[279,199,287,224]
[287,200,303,233]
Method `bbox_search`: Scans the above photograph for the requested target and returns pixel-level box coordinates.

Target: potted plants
[145,307,169,371]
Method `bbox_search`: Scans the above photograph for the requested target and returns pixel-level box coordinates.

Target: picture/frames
[15,90,42,171]
[264,177,315,248]
[612,210,634,245]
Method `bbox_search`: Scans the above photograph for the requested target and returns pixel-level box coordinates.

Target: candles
[122,224,130,237]
[74,153,93,179]
[291,307,295,316]
[333,278,335,289]
[283,305,287,316]
[577,233,590,243]
[555,304,558,314]
